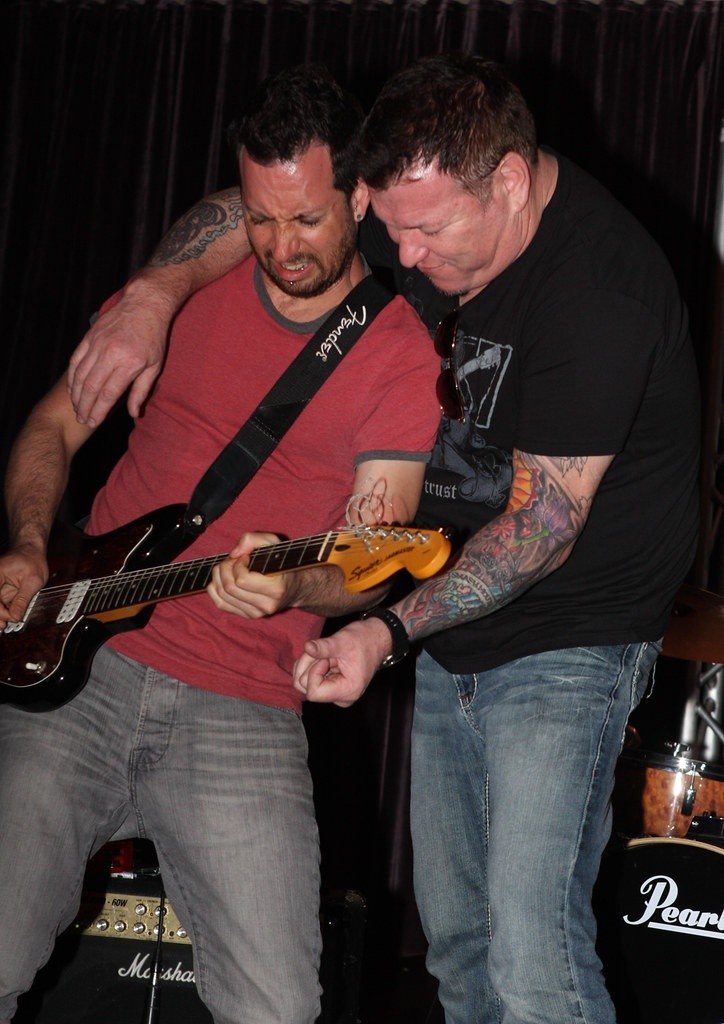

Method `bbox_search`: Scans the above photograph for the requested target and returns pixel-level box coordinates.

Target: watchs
[359,605,412,667]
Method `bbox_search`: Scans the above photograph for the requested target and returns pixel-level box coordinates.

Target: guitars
[1,475,456,714]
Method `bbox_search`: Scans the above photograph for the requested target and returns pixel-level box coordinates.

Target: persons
[0,66,445,1024]
[293,48,701,1024]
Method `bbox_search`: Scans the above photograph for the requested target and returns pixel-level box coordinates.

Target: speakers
[9,876,214,1024]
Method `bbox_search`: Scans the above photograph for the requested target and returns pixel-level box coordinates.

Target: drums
[639,743,724,842]
